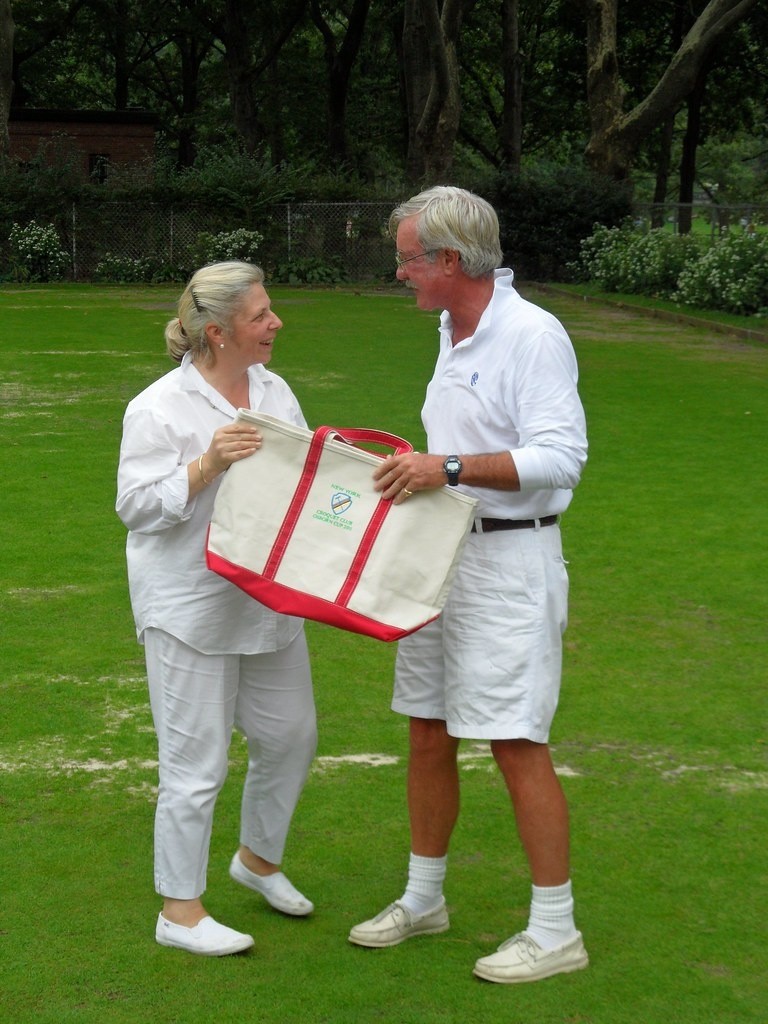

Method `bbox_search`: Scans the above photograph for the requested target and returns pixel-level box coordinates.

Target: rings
[404,489,412,497]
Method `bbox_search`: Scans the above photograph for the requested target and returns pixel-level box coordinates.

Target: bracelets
[199,455,214,485]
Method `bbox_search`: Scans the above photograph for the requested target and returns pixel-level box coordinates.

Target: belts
[471,514,557,533]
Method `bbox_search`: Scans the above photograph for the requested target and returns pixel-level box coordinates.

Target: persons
[115,263,315,958]
[348,185,589,984]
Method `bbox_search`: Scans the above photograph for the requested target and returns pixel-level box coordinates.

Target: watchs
[444,455,462,486]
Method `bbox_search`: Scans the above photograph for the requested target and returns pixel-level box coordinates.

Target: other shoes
[472,930,589,983]
[348,895,449,946]
[154,910,254,957]
[229,850,314,915]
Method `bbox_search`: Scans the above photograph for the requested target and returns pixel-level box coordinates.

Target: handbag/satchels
[205,408,482,641]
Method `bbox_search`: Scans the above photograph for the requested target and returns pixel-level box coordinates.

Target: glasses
[395,249,440,270]
